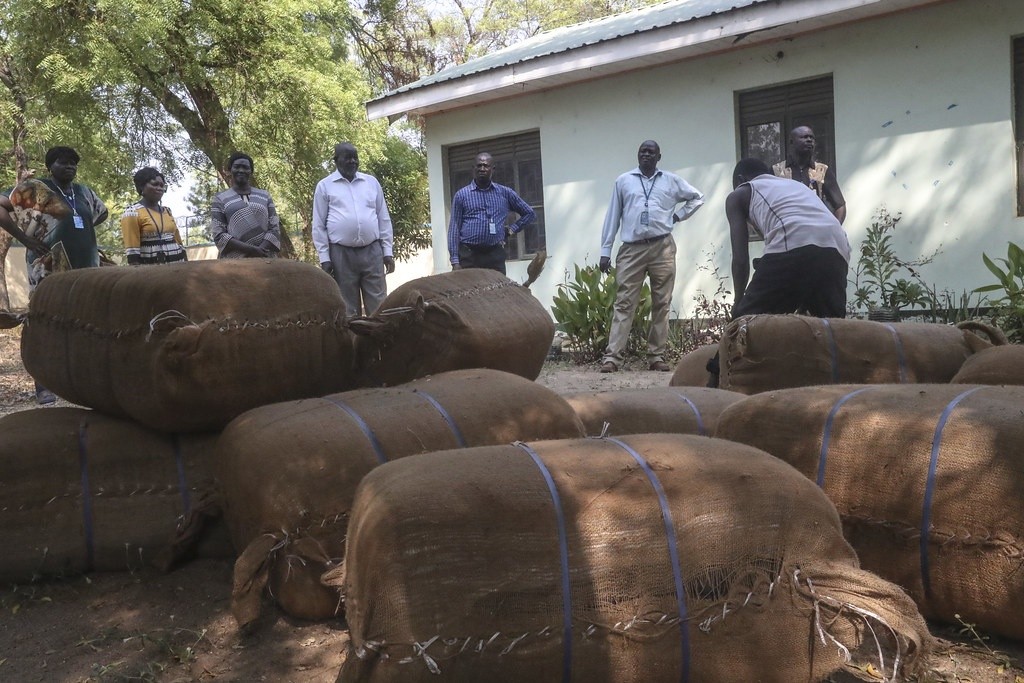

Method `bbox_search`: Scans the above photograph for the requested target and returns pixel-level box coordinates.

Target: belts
[634,234,669,245]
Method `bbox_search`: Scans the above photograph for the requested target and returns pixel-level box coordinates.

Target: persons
[211,153,281,258]
[724,157,852,321]
[447,152,535,275]
[312,142,397,315]
[121,167,188,265]
[0,147,109,404]
[600,140,704,372]
[769,125,846,224]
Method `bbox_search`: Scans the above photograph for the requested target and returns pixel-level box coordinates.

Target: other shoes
[601,361,618,373]
[650,361,670,372]
[36,391,54,404]
